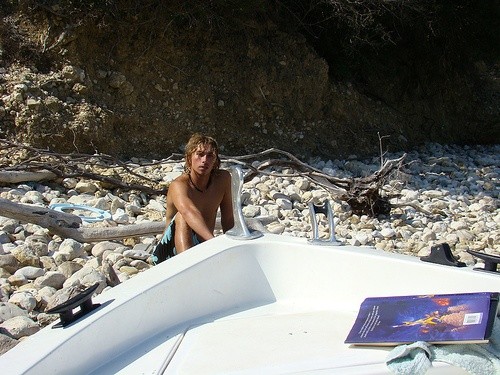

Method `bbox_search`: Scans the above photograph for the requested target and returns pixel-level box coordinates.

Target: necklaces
[187,172,213,193]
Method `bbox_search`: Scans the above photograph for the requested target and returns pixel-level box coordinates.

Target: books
[343,291,500,345]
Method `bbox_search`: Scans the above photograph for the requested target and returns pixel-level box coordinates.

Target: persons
[152,134,235,264]
